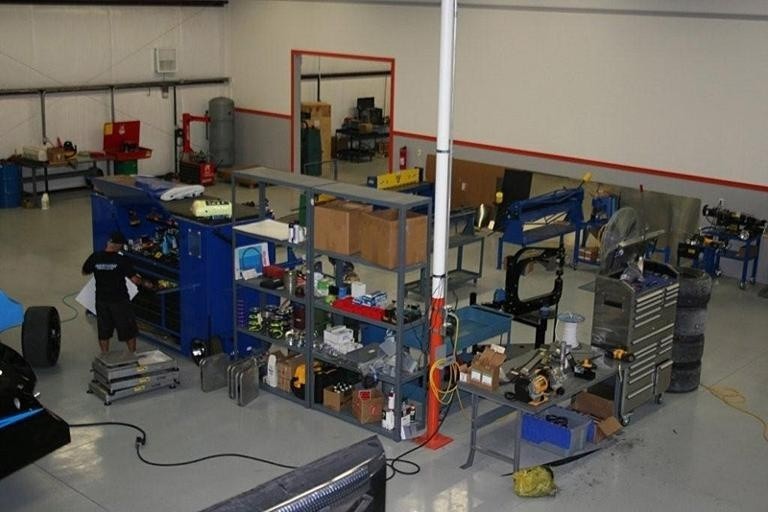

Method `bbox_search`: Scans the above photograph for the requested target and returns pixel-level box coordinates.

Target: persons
[81,230,141,352]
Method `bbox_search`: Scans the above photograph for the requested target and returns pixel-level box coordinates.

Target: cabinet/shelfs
[230,166,434,442]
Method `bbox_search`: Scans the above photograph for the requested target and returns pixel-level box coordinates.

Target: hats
[108,233,128,245]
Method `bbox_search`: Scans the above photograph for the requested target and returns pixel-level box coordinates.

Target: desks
[334,127,378,164]
[456,341,626,485]
[14,152,101,209]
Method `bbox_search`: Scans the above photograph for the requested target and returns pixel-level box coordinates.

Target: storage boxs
[521,394,622,460]
[312,199,373,255]
[323,382,354,411]
[351,389,383,424]
[361,209,428,271]
[504,255,535,276]
